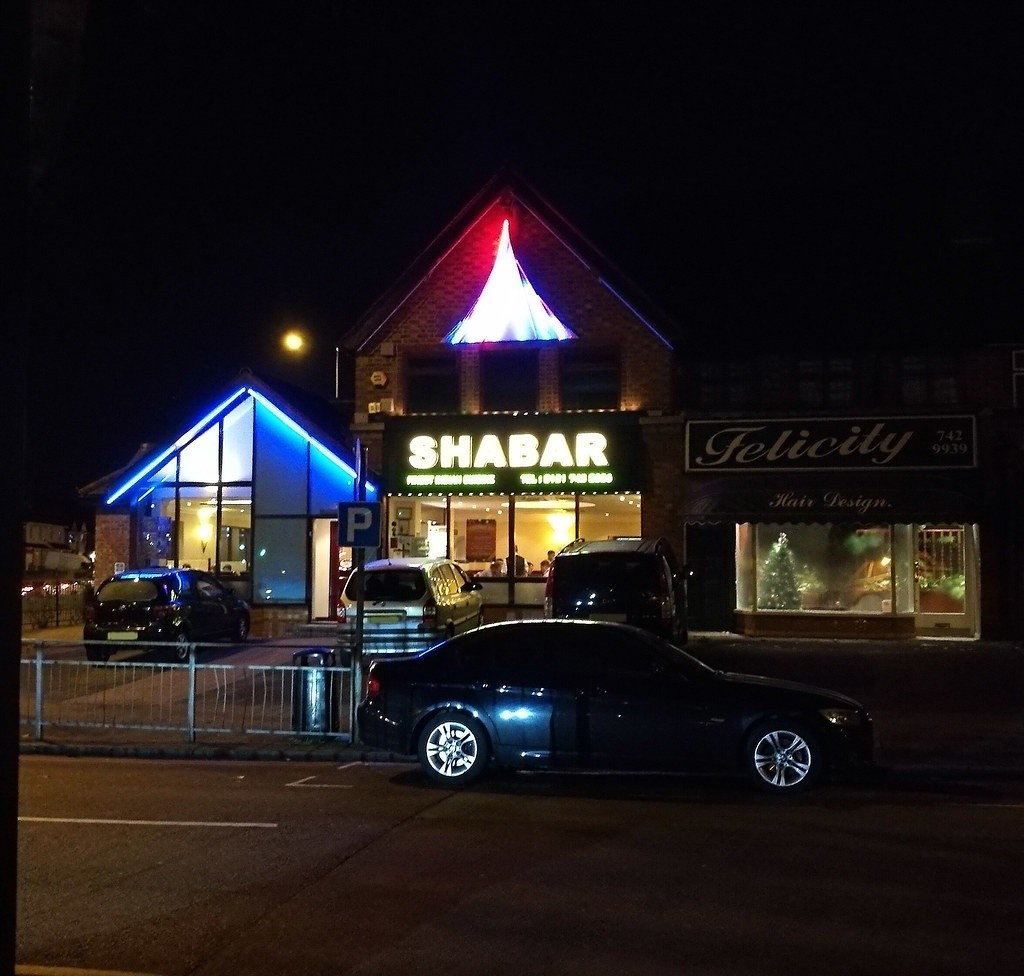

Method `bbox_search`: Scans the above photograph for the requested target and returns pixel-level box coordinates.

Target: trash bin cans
[290,646,341,747]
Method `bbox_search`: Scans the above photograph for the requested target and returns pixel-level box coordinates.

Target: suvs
[544,538,695,645]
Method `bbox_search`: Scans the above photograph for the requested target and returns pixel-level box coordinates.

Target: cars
[359,620,875,791]
[338,558,484,668]
[82,566,254,663]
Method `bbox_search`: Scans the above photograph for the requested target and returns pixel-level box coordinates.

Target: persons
[481,542,555,580]
